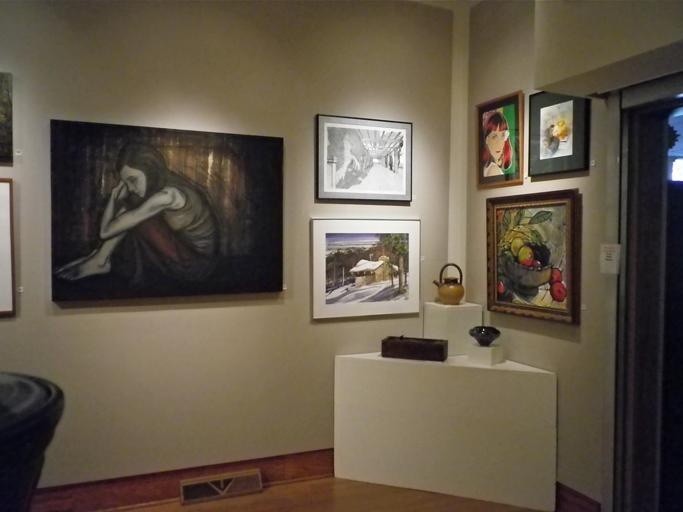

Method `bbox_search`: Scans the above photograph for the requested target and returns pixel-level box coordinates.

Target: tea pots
[432,262,465,306]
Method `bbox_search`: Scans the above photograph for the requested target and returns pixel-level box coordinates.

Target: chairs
[1,370,66,512]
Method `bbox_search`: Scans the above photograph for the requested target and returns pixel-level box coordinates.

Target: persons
[50,140,222,286]
[482,110,514,178]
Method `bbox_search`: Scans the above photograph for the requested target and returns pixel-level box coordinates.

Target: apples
[550,282,566,301]
[549,268,563,286]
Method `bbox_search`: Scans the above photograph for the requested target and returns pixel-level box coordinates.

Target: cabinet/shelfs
[335,350,557,512]
[422,302,482,355]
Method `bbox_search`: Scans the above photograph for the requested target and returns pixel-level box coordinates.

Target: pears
[518,246,534,266]
[511,238,524,257]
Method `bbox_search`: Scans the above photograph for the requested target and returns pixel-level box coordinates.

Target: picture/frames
[473,85,591,326]
[0,178,16,323]
[307,113,421,320]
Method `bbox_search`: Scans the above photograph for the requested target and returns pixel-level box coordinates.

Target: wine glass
[504,256,552,297]
